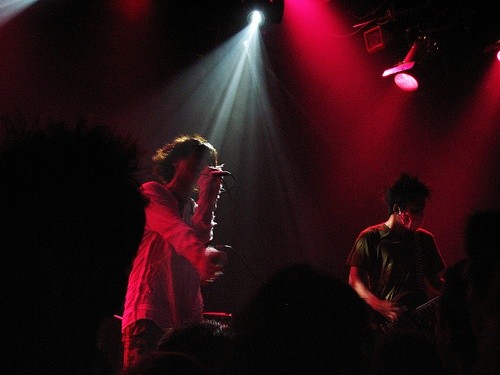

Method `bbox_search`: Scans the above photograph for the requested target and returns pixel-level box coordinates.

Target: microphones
[209,171,230,177]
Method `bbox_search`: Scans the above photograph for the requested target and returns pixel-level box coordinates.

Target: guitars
[358,295,441,343]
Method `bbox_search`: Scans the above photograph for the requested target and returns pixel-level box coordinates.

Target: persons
[347,172,468,375]
[123,320,237,375]
[1,111,145,375]
[118,134,229,375]
[375,330,445,375]
[232,264,367,375]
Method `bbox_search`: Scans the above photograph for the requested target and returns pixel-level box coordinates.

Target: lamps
[393,33,439,92]
